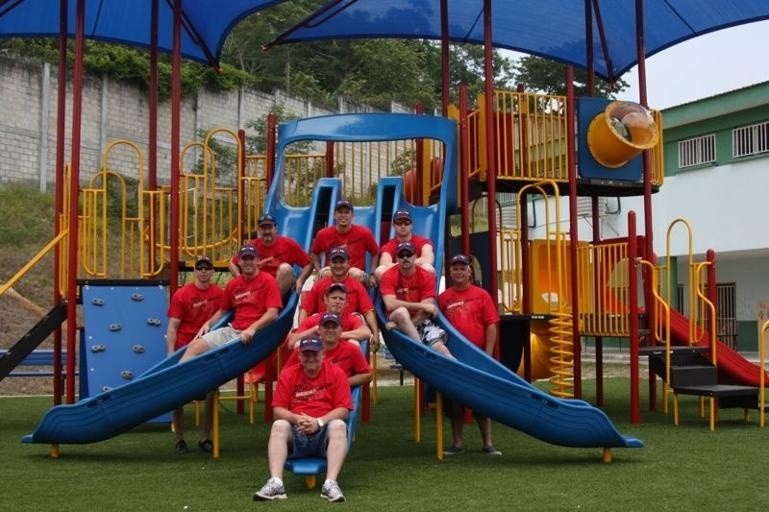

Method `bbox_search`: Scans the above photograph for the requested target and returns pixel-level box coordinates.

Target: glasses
[195,265,213,270]
[395,222,410,226]
[398,253,414,258]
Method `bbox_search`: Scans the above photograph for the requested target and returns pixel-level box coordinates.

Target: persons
[228,214,314,297]
[287,283,372,351]
[380,242,456,360]
[253,334,354,502]
[437,255,500,455]
[166,256,222,455]
[310,201,381,288]
[298,247,380,353]
[280,313,373,387]
[374,208,437,281]
[178,244,283,363]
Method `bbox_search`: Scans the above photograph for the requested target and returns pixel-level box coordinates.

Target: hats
[334,200,354,212]
[451,254,469,265]
[258,214,276,226]
[393,210,412,222]
[396,242,415,254]
[299,334,323,352]
[330,247,348,261]
[240,245,257,259]
[196,256,212,267]
[326,283,347,296]
[321,312,340,325]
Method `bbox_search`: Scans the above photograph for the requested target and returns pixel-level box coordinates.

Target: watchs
[315,418,323,430]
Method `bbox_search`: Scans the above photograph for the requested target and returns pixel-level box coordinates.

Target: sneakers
[199,440,223,453]
[253,480,288,501]
[482,447,502,457]
[320,484,345,502]
[443,446,466,457]
[175,440,188,455]
